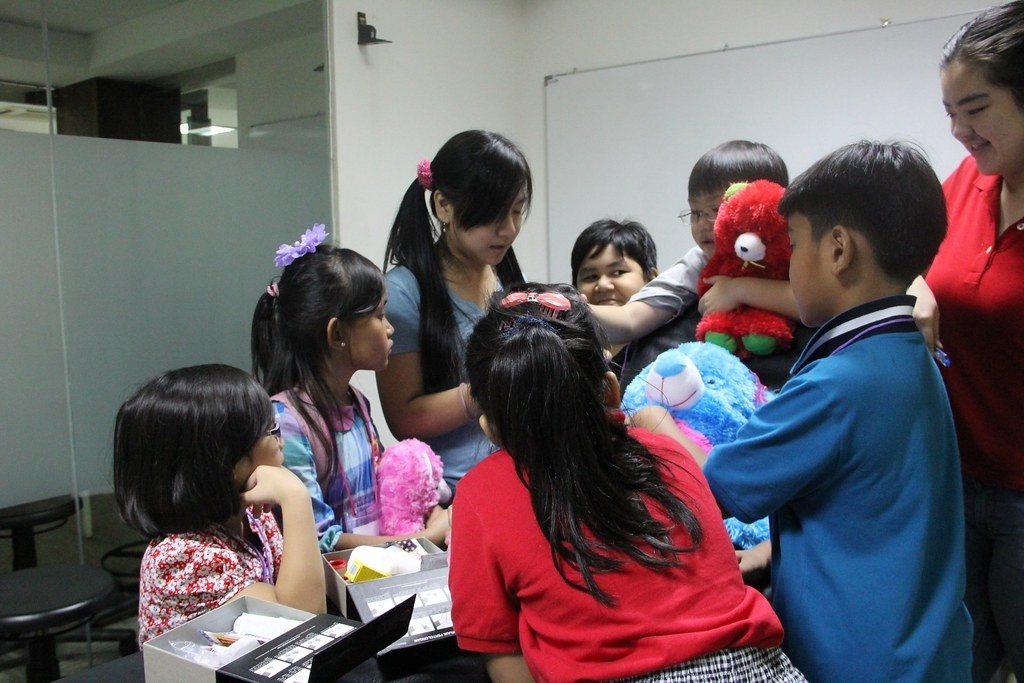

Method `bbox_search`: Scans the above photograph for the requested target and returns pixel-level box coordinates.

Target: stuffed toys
[618,343,778,549]
[377,438,451,538]
[696,178,794,358]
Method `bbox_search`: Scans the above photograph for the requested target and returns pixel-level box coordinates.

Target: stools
[0,564,139,683]
[0,494,83,570]
[101,537,148,578]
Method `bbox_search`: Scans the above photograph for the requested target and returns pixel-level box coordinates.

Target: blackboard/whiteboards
[541,8,1013,287]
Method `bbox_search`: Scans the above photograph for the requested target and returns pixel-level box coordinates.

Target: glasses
[263,421,282,439]
[678,203,720,224]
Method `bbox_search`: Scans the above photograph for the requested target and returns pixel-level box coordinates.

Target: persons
[631,141,975,683]
[578,140,819,403]
[114,364,328,651]
[251,245,452,553]
[373,128,533,500]
[570,219,658,380]
[905,1,1024,683]
[446,283,809,683]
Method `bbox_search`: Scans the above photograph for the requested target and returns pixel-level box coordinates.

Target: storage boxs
[143,594,417,683]
[322,537,454,662]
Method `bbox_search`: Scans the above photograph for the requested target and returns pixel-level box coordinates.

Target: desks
[51,621,493,683]
